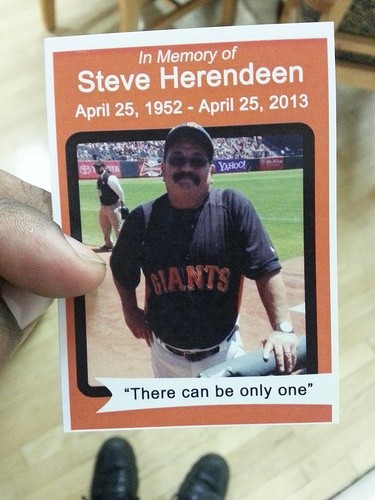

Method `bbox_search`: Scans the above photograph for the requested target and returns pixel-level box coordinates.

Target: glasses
[163,155,209,167]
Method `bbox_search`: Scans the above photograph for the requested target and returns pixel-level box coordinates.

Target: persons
[211,136,298,159]
[110,122,298,378]
[0,192,230,499]
[92,159,129,249]
[76,140,165,160]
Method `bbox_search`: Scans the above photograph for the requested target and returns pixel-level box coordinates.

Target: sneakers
[100,243,114,252]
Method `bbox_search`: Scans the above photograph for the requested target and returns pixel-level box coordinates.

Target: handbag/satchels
[121,200,129,219]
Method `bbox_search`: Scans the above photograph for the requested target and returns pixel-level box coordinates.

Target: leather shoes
[176,453,230,500]
[89,437,139,500]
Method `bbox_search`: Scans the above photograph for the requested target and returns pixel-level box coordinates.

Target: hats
[163,122,215,161]
[93,158,104,166]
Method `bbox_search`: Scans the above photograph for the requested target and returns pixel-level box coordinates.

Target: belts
[153,326,236,362]
